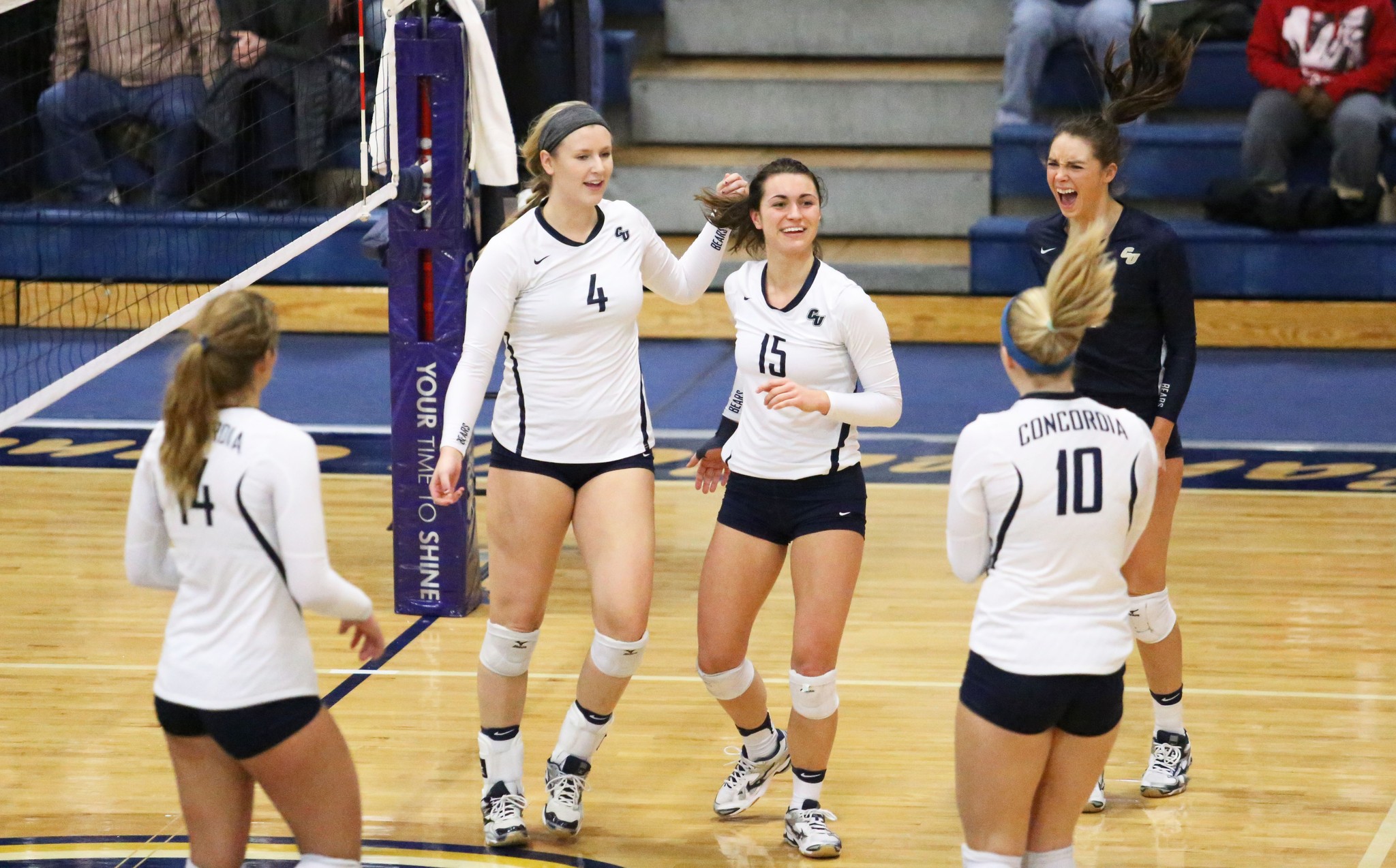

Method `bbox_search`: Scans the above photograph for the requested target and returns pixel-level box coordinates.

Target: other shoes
[194,175,243,207]
[267,178,320,212]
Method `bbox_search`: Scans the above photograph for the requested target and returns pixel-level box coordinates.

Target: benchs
[0,0,640,283]
[967,0,1396,300]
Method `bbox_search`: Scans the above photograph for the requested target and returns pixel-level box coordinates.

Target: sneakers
[713,729,791,817]
[482,779,531,846]
[1082,769,1106,811]
[1141,729,1193,797]
[783,805,842,857]
[541,757,592,837]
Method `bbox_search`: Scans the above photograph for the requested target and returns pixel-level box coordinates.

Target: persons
[947,219,1159,867]
[686,159,905,861]
[37,0,380,212]
[489,0,557,74]
[1026,14,1197,814]
[123,291,385,868]
[1239,1,1396,198]
[995,0,1154,125]
[428,101,749,848]
